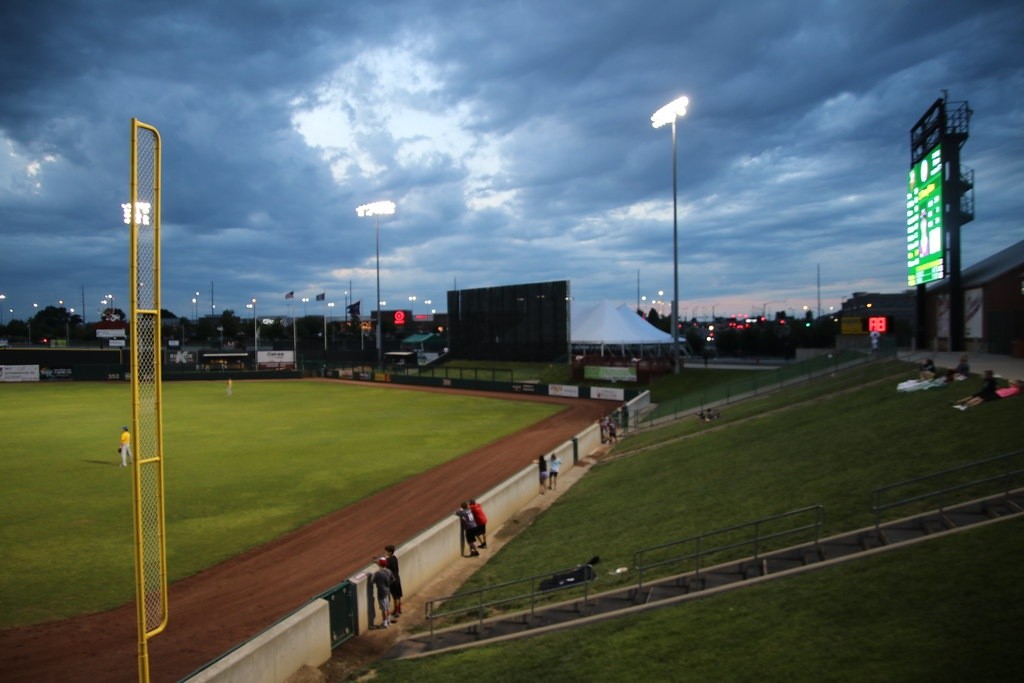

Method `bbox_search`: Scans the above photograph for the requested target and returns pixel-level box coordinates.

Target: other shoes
[392,612,402,617]
[376,623,392,628]
[470,551,480,557]
[953,405,967,411]
[478,543,487,548]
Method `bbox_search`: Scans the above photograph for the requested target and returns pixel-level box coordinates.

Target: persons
[699,409,714,424]
[952,370,999,411]
[943,354,970,384]
[225,378,232,400]
[371,545,403,629]
[919,358,935,380]
[119,426,133,467]
[455,499,487,557]
[600,402,629,444]
[532,454,562,495]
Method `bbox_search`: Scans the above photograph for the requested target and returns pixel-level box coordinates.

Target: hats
[377,557,388,566]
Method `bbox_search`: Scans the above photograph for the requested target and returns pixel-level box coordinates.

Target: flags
[286,292,294,299]
[316,293,325,301]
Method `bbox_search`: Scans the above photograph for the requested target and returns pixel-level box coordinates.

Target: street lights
[409,296,416,311]
[425,299,431,319]
[29,304,37,345]
[191,292,200,330]
[121,201,152,349]
[356,199,396,365]
[650,96,690,369]
[328,303,334,343]
[252,299,258,371]
[108,295,112,310]
[59,301,69,346]
[302,298,309,320]
